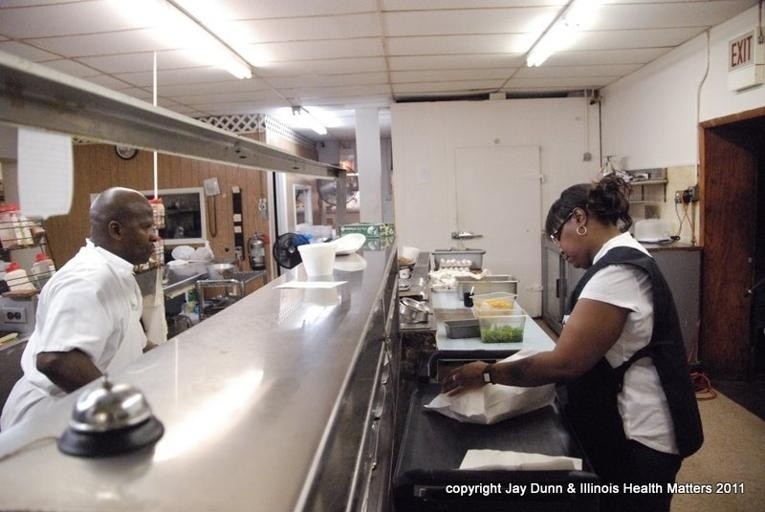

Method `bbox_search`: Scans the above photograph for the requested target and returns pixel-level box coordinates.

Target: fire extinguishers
[247,232,268,270]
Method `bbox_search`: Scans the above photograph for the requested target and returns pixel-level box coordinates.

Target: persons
[1,186,162,438]
[439,171,705,512]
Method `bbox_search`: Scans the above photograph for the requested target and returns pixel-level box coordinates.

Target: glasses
[550,212,575,248]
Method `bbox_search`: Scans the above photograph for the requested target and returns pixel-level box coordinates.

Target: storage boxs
[471,305,529,345]
[468,291,518,313]
[442,318,478,337]
[454,274,521,303]
[432,248,488,271]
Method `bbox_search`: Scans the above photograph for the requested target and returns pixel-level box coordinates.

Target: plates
[334,253,367,272]
[330,232,367,256]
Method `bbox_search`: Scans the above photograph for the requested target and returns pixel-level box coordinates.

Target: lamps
[521,1,591,69]
[160,0,254,86]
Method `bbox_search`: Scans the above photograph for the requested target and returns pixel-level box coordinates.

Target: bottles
[0,204,57,294]
[149,198,166,265]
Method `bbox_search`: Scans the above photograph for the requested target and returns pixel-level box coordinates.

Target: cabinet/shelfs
[194,268,266,321]
[538,227,704,371]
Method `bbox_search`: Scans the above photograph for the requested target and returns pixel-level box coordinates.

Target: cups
[463,292,475,306]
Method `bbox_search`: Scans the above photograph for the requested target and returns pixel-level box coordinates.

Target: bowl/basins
[399,296,429,324]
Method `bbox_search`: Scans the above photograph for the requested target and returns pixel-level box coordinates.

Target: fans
[314,160,360,210]
[271,229,309,270]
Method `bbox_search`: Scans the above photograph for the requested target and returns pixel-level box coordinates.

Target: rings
[451,373,456,382]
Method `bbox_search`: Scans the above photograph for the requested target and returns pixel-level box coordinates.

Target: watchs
[482,362,496,387]
